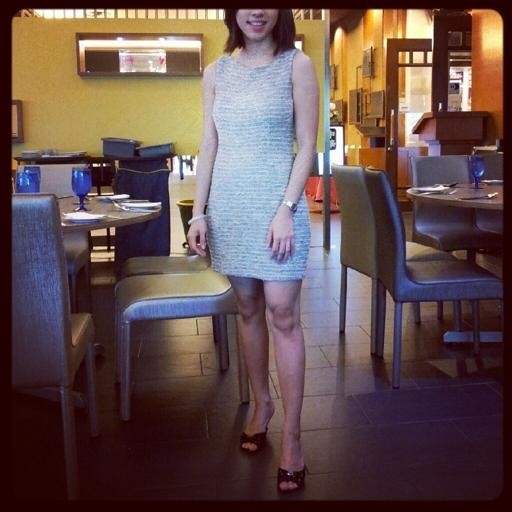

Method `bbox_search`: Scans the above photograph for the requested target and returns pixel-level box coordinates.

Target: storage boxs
[102,139,140,161]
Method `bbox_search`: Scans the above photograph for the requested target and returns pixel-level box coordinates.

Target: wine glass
[71,165,91,212]
[467,155,486,190]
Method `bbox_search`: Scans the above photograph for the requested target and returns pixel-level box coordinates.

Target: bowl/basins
[21,150,45,159]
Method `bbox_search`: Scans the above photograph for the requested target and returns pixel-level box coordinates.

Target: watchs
[282,198,297,214]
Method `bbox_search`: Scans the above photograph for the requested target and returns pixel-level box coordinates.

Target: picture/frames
[12,100,23,142]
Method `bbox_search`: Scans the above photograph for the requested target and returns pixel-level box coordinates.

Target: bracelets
[186,214,212,225]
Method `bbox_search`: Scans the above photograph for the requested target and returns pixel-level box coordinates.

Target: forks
[418,189,460,196]
[458,191,499,200]
[430,181,460,187]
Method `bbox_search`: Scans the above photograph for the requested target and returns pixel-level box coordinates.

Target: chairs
[12,158,256,496]
[328,157,501,392]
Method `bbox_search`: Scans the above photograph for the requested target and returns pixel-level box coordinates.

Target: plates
[60,212,103,227]
[86,191,163,215]
[480,179,503,186]
[410,184,451,193]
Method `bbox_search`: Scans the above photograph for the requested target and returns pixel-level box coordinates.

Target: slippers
[240,426,269,453]
[277,463,307,494]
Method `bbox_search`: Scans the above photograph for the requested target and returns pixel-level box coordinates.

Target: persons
[186,8,323,495]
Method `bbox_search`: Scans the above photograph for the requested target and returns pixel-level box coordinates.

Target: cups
[15,164,42,194]
